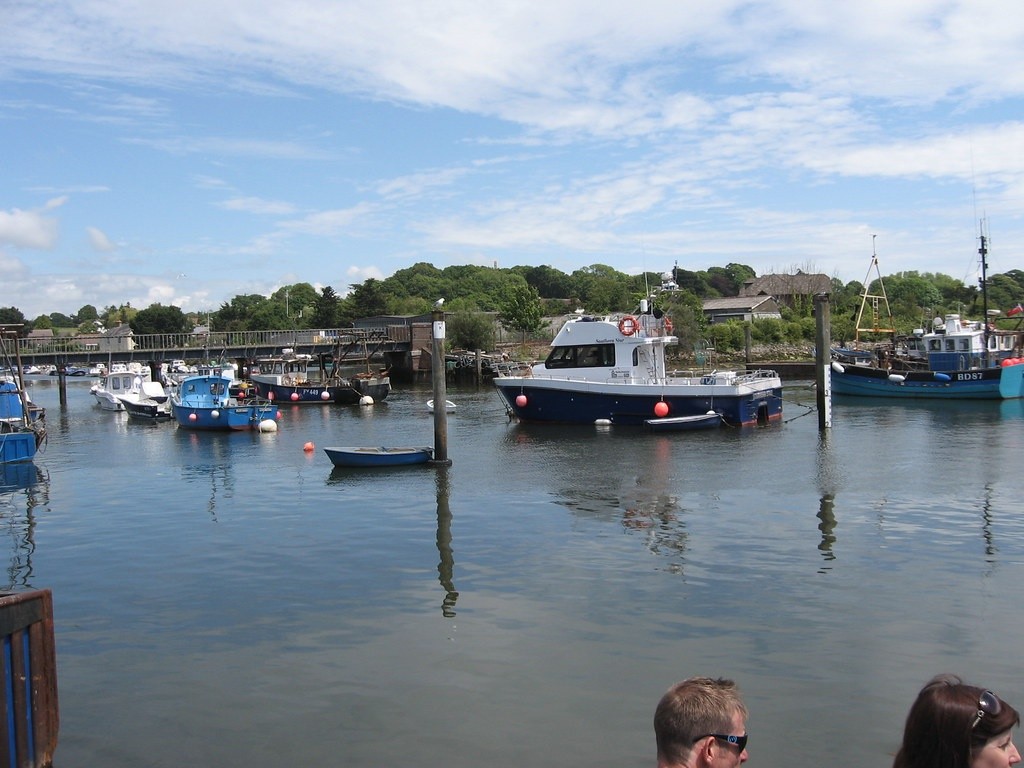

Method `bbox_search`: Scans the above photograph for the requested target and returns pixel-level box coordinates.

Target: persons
[654,676,749,768]
[893,673,1021,768]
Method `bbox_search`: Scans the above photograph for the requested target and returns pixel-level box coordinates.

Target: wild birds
[178,272,189,280]
[432,298,446,311]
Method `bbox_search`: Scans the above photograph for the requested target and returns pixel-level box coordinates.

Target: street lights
[206,309,213,346]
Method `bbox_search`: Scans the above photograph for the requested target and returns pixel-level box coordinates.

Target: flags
[1006,306,1023,318]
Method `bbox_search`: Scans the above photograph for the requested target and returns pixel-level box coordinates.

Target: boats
[0,323,48,464]
[0,461,50,590]
[90,368,107,376]
[323,446,434,465]
[172,346,277,432]
[427,398,458,413]
[325,465,433,486]
[164,366,255,399]
[116,381,172,419]
[249,330,396,404]
[813,220,1023,400]
[89,351,152,411]
[492,313,783,431]
[10,365,86,375]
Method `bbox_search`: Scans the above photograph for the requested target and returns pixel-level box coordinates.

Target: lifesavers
[665,316,672,331]
[619,316,638,336]
[282,374,291,387]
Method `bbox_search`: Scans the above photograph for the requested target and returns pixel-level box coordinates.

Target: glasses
[694,733,747,753]
[971,690,1001,728]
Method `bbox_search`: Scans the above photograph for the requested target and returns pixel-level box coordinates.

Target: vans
[171,360,186,367]
